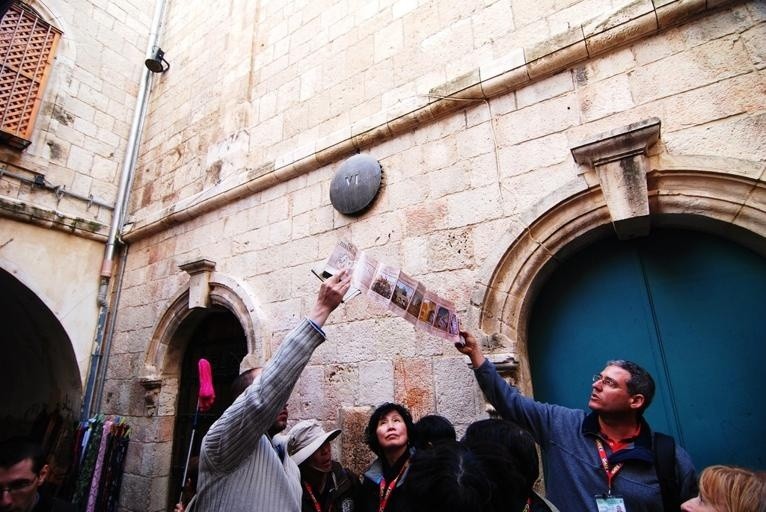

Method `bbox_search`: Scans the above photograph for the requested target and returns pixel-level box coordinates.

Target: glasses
[0,473,37,491]
[593,375,627,392]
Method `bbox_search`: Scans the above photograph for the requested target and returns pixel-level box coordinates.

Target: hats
[287,419,342,465]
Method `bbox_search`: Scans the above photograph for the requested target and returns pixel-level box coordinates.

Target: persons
[680,464,766,511]
[0,437,51,512]
[286,403,560,511]
[455,329,699,511]
[194,269,351,512]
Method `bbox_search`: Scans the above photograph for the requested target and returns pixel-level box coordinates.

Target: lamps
[145,44,169,73]
